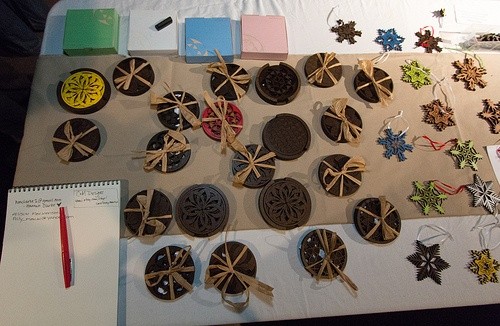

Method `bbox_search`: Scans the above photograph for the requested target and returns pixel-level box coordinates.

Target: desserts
[52,8,496,311]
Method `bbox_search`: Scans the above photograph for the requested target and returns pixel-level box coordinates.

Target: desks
[1,0,500,326]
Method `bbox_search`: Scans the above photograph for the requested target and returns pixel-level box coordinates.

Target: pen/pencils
[60,207,71,288]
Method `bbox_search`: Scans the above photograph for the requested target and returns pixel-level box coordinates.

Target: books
[1,178,124,326]
